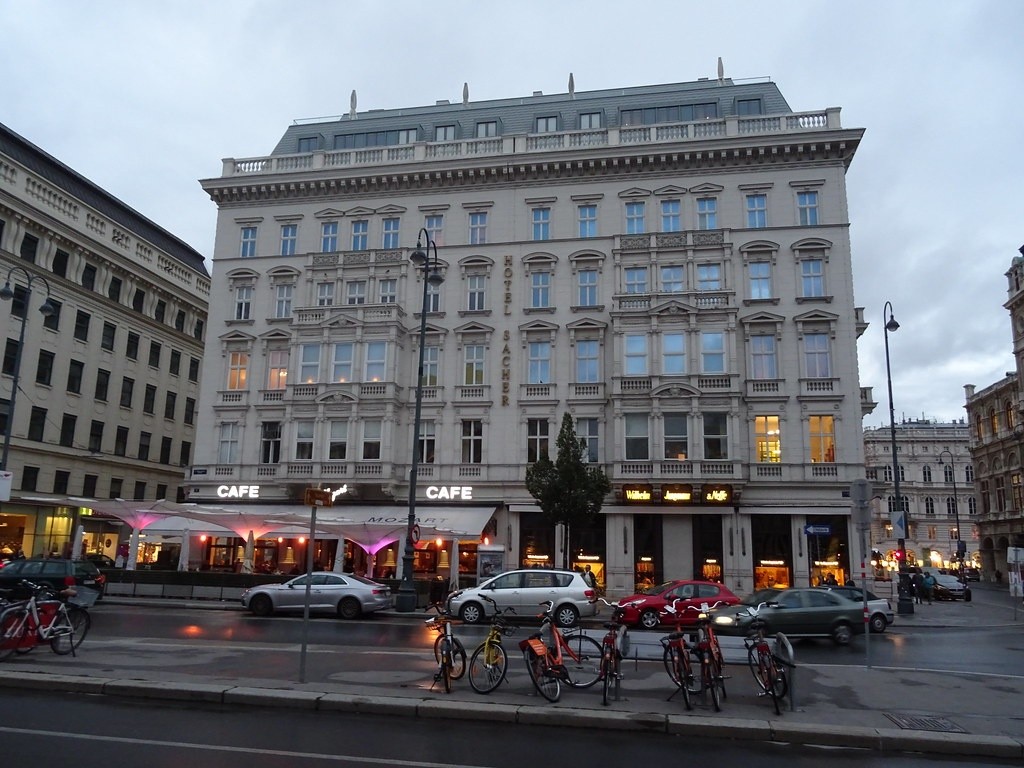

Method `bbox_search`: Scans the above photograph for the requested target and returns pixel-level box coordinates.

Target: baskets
[67,586,100,609]
[425,609,456,631]
[562,619,584,633]
[492,615,520,637]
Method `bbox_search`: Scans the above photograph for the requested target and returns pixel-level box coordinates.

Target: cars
[895,567,980,602]
[445,570,599,628]
[612,580,894,647]
[241,572,394,621]
[83,553,127,568]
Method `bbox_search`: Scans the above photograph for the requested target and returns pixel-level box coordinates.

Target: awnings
[141,505,497,535]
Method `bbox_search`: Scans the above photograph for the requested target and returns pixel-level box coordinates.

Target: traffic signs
[804,525,831,535]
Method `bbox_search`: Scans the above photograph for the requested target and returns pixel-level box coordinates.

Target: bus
[871,548,885,572]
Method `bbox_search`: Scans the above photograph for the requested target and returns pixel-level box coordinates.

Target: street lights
[938,451,967,586]
[1,266,55,470]
[394,228,446,611]
[884,302,914,614]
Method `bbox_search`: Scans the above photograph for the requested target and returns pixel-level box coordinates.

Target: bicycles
[518,600,604,703]
[679,600,731,712]
[424,592,467,694]
[656,597,711,711]
[469,593,519,695]
[735,601,785,716]
[0,578,100,663]
[589,597,642,705]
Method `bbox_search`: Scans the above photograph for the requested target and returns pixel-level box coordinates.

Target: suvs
[0,557,106,608]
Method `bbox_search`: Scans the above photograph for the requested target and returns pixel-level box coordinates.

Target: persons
[269,560,278,572]
[234,558,241,572]
[120,545,128,567]
[82,539,88,557]
[582,563,596,588]
[288,564,301,575]
[11,548,26,562]
[912,567,937,606]
[817,570,855,586]
[386,566,396,579]
[313,562,324,571]
